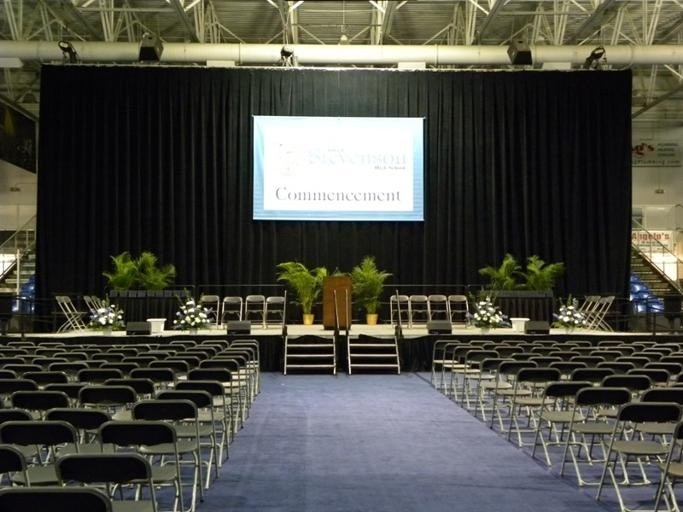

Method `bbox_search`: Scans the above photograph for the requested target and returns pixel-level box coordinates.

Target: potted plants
[349,257,393,325]
[276,260,330,324]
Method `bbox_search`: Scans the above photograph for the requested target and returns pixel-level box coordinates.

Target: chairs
[390,295,472,329]
[55,294,105,331]
[576,295,615,333]
[196,295,286,329]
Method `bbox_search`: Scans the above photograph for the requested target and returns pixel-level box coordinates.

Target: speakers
[140,34,163,61]
[428,320,451,333]
[126,322,151,335]
[227,320,251,334]
[525,321,549,334]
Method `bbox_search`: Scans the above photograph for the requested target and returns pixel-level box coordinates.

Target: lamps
[138,35,164,63]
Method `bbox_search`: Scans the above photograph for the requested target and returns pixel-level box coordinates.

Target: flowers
[171,287,210,327]
[551,293,588,329]
[469,289,503,327]
[85,304,126,332]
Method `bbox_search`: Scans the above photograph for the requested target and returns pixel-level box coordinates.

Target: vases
[103,329,112,337]
[482,327,490,335]
[190,328,197,335]
